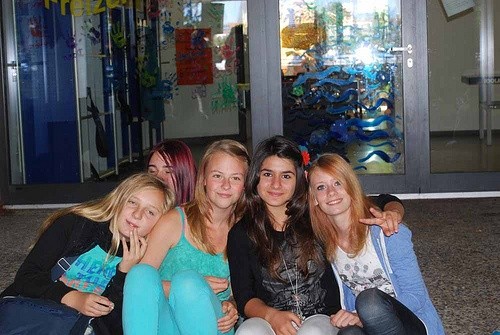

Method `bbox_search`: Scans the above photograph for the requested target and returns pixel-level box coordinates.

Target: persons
[122,140,250,335]
[227,135,405,335]
[143,138,197,208]
[0,172,175,334]
[308,153,445,335]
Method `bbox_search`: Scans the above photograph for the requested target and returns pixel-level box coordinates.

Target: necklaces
[275,233,306,322]
